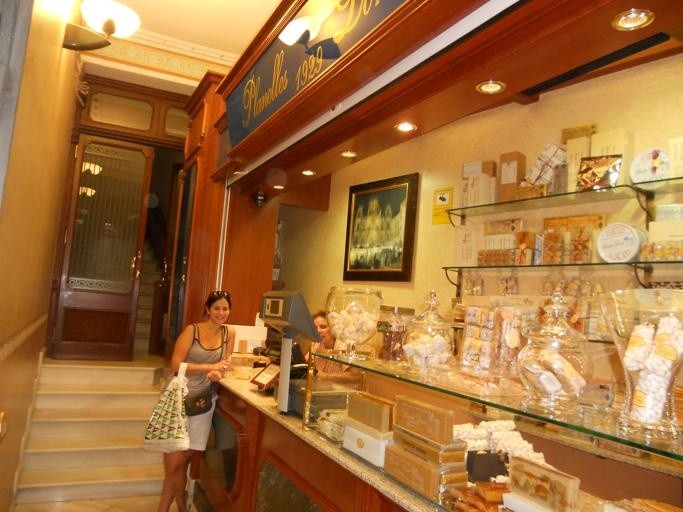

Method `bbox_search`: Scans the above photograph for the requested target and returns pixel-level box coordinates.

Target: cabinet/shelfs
[300,176,683,462]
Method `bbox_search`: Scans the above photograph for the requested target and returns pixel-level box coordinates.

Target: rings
[223,366,227,370]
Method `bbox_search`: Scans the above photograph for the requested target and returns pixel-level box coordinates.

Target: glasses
[208,289,231,300]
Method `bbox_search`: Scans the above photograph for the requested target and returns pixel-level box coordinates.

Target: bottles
[517,287,594,408]
[402,291,454,375]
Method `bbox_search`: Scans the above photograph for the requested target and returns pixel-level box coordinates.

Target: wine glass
[323,285,382,362]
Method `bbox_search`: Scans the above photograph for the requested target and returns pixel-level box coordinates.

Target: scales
[260,289,357,419]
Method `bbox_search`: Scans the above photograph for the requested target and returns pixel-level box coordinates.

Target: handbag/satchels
[183,390,214,417]
[138,360,193,454]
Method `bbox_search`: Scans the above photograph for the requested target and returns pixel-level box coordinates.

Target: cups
[600,289,683,443]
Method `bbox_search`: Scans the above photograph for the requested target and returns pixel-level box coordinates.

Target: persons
[303,307,353,367]
[156,288,237,511]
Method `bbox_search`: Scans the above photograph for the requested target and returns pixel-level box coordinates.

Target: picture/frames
[343,172,421,282]
